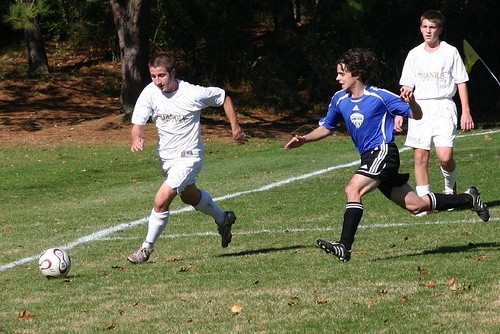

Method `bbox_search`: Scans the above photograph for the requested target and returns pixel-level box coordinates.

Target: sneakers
[316,239,352,263]
[464,186,491,222]
[127,247,154,265]
[214,211,237,248]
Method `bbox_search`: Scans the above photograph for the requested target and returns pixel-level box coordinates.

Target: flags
[462,40,478,73]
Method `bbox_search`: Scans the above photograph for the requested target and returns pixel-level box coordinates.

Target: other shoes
[443,182,459,212]
[411,209,434,218]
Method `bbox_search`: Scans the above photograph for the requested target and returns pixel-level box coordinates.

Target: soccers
[38,247,73,279]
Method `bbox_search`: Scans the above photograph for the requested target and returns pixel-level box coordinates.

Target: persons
[127,52,250,264]
[399,11,474,216]
[285,51,491,263]
